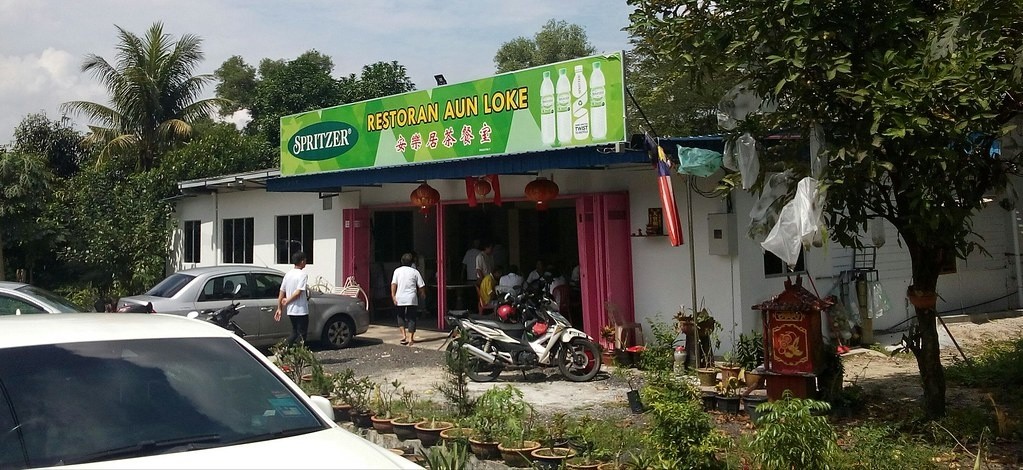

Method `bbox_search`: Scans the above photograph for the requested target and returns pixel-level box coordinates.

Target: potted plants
[272,295,768,470]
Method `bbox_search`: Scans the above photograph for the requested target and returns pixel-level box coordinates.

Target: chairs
[552,283,574,325]
[474,284,499,318]
[223,280,234,299]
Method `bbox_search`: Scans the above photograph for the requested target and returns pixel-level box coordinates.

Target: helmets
[497,305,516,321]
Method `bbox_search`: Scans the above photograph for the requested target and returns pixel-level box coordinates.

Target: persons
[476,241,494,314]
[499,265,524,287]
[542,268,566,294]
[479,266,503,319]
[460,240,482,284]
[391,251,425,346]
[526,260,552,284]
[274,252,309,363]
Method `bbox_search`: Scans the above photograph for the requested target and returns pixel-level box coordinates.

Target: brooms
[806,269,851,354]
[933,306,1021,437]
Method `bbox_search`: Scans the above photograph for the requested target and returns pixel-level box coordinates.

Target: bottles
[556,69,572,141]
[540,72,555,144]
[590,62,607,138]
[572,65,589,140]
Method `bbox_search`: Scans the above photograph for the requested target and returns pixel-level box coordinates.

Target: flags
[658,146,684,247]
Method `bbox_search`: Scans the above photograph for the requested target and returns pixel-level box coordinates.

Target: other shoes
[410,340,414,343]
[275,351,285,364]
[400,338,405,341]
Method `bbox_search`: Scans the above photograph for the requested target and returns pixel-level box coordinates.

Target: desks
[418,287,465,319]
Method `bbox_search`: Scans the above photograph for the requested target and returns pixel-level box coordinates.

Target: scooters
[112,284,249,340]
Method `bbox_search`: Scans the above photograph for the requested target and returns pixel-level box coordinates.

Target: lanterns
[410,184,440,214]
[475,180,491,197]
[525,177,559,210]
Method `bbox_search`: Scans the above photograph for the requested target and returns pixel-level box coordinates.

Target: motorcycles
[437,271,600,382]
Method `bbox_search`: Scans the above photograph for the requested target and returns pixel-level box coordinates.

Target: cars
[0,281,89,316]
[0,313,432,470]
[116,264,370,351]
